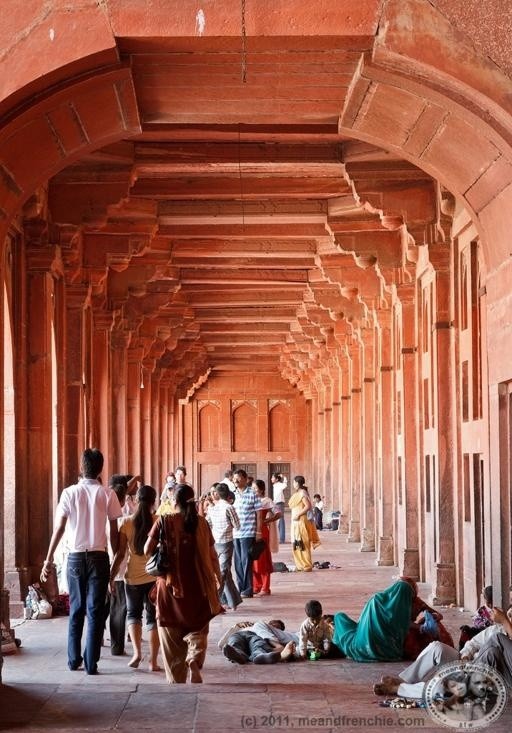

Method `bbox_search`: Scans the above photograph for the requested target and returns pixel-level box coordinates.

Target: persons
[435,671,498,722]
[40,448,512,702]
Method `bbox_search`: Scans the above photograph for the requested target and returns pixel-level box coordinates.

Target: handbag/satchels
[144,542,166,576]
[249,540,264,560]
